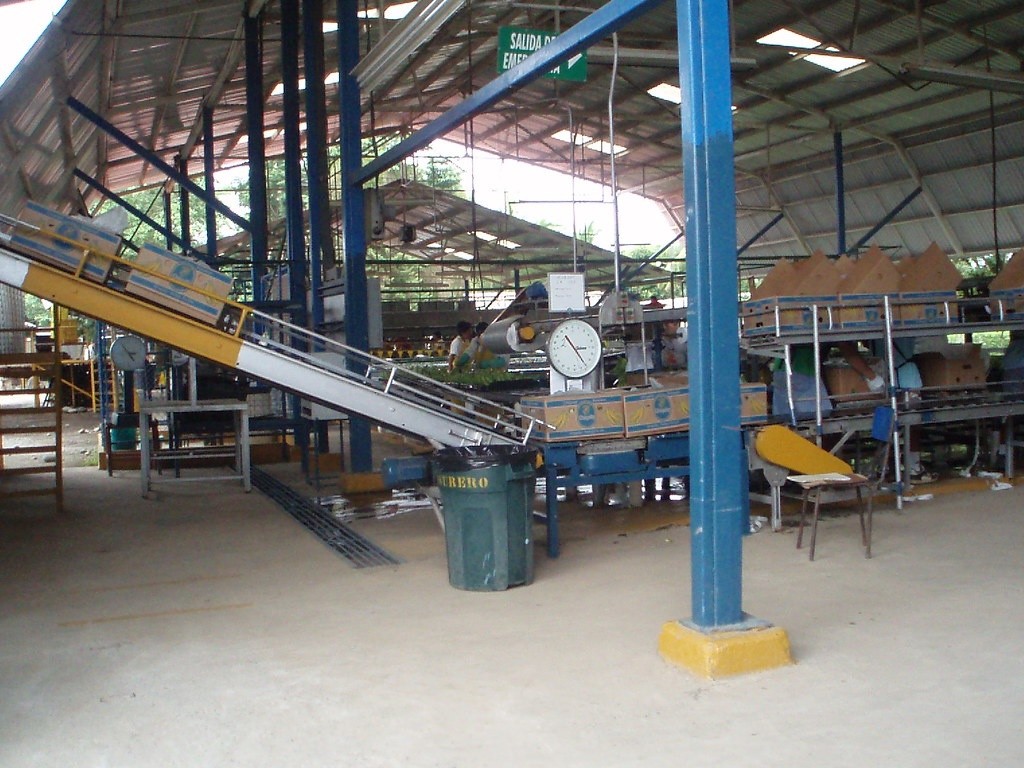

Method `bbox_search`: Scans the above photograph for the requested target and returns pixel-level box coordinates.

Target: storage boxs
[743,295,841,338]
[838,292,901,328]
[823,366,882,416]
[989,287,1024,320]
[124,241,234,328]
[920,355,988,399]
[741,381,767,425]
[519,392,626,442]
[600,382,691,440]
[7,199,123,286]
[900,291,959,327]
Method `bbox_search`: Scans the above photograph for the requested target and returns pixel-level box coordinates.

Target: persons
[772,337,939,483]
[643,297,663,309]
[430,319,511,374]
[658,321,687,368]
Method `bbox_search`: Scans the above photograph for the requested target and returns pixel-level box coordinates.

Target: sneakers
[910,467,938,484]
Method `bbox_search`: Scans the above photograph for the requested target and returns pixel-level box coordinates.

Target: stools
[106,419,162,475]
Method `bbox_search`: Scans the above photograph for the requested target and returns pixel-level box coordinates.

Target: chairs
[792,406,897,562]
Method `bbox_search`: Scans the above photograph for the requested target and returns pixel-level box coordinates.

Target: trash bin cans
[433,444,542,594]
[111,426,136,450]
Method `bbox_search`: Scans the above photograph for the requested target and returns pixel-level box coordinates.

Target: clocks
[548,317,603,381]
[109,334,147,372]
